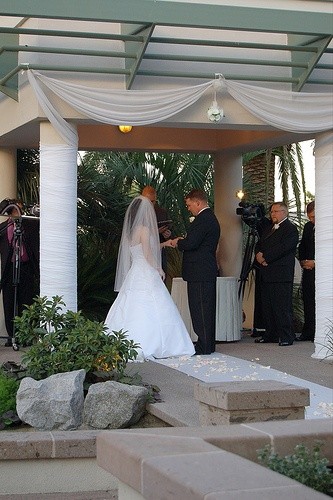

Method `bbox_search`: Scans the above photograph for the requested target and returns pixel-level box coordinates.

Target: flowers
[189,217,195,222]
[207,107,223,123]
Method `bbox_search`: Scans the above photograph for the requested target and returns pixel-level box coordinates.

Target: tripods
[239,225,261,299]
[0,219,40,351]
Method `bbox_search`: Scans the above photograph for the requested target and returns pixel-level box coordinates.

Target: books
[157,220,173,227]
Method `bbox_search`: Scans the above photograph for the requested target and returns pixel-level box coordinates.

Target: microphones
[239,202,251,208]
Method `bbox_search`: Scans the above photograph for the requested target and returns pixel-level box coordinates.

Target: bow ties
[189,217,195,222]
[272,224,280,230]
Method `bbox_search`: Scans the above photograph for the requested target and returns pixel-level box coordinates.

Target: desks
[170,277,243,344]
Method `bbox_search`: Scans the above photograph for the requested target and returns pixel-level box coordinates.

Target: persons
[0,199,40,347]
[101,186,315,361]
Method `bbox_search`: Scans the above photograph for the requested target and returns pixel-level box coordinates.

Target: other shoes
[295,336,310,341]
[255,338,266,343]
[279,341,293,346]
